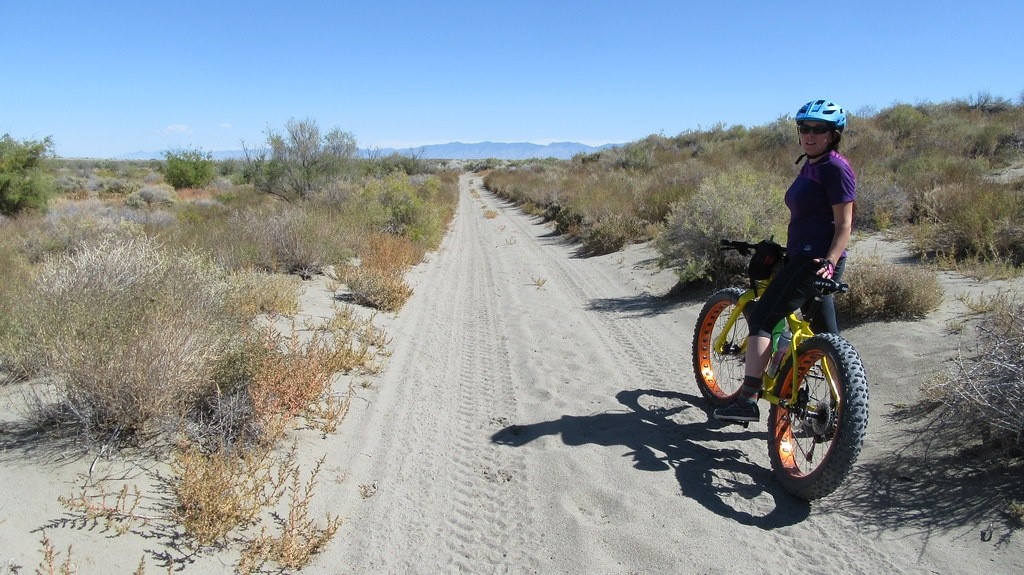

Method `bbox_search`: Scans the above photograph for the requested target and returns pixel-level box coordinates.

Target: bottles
[765,326,793,380]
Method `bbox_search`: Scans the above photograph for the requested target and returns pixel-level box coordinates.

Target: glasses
[798,125,833,134]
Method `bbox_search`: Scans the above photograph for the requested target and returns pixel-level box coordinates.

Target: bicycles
[690,236,869,503]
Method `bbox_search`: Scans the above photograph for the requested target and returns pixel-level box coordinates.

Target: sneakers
[713,399,760,422]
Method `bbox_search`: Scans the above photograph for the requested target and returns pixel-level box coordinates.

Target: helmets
[795,99,846,132]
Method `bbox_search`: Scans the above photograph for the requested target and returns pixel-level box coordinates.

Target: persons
[706,101,859,441]
